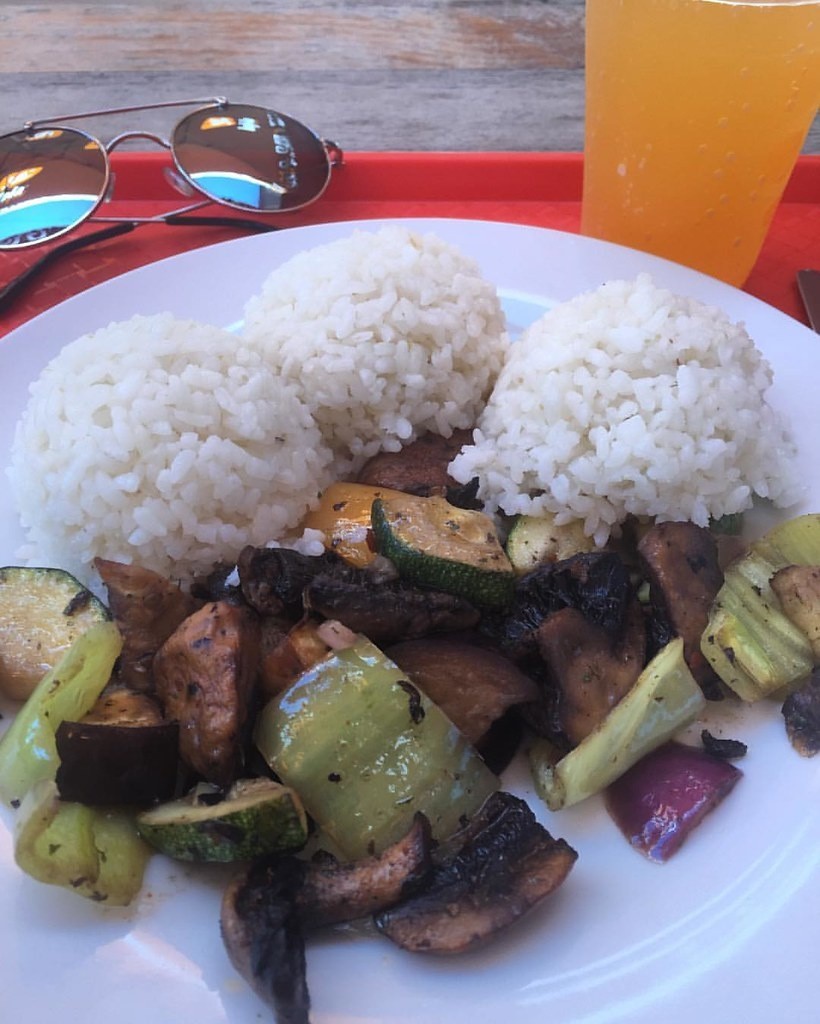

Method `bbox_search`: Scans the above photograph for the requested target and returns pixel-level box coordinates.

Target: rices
[443,272,808,549]
[6,310,331,583]
[238,230,507,472]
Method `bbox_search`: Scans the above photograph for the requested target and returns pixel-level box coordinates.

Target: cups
[579,0,820,289]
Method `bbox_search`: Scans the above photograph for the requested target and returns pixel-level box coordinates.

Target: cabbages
[702,511,820,703]
[0,621,158,910]
[527,637,704,812]
[251,632,503,860]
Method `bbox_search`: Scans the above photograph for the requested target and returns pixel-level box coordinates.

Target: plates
[0,218,819,1024]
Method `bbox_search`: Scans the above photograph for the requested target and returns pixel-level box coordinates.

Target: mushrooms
[54,423,820,1024]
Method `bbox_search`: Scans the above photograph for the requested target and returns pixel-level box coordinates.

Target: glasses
[0,97,345,313]
